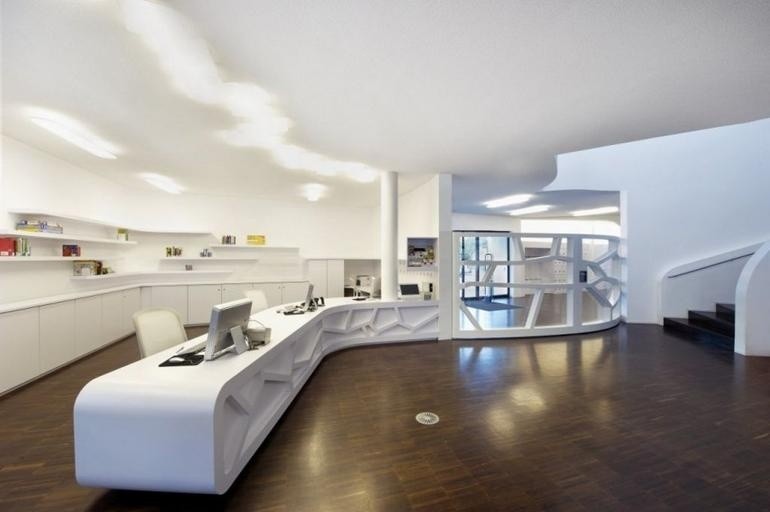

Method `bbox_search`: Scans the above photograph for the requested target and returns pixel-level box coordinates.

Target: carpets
[464,298,523,311]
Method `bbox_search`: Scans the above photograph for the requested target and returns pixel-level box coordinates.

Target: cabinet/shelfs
[0,209,301,280]
[253,284,309,314]
[189,285,252,324]
[0,287,151,398]
[151,285,189,325]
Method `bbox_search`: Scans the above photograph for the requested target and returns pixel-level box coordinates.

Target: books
[0,235,31,257]
[63,244,113,276]
[15,220,64,234]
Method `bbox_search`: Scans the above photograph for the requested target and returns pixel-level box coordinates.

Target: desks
[73,294,439,495]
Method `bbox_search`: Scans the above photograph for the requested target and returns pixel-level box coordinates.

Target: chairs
[245,289,268,314]
[134,306,188,359]
